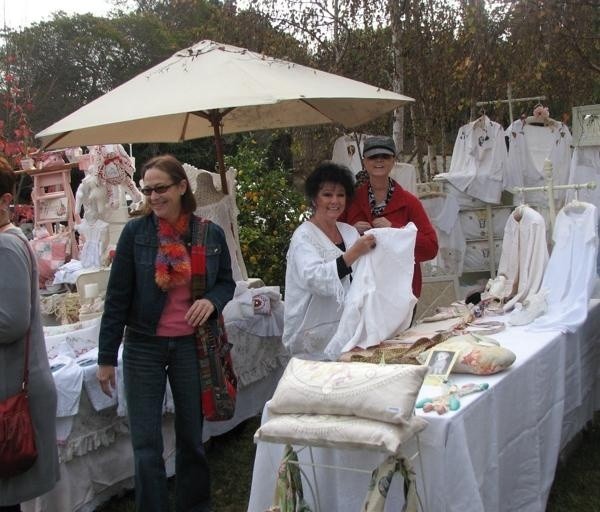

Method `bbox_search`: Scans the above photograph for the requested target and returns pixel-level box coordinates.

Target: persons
[337,137,441,331]
[0,158,61,511]
[188,172,250,284]
[283,161,379,362]
[97,154,235,510]
[74,143,144,268]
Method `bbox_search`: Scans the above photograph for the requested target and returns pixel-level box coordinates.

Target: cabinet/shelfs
[30,169,84,263]
[431,171,542,281]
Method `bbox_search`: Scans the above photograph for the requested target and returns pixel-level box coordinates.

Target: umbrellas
[32,39,417,197]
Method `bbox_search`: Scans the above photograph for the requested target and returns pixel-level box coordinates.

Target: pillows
[30,232,71,290]
[252,355,429,455]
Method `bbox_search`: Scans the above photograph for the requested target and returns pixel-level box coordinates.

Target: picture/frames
[424,346,461,379]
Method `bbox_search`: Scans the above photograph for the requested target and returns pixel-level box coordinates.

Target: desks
[21,300,290,512]
[264,297,599,512]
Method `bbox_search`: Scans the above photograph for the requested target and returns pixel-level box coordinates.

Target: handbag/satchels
[198,314,238,422]
[0,391,39,481]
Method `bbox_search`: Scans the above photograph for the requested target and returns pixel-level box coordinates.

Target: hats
[363,135,397,159]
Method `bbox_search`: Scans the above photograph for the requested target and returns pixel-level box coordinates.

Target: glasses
[141,182,178,196]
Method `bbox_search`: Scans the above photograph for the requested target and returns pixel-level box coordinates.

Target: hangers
[463,99,566,131]
[507,189,596,226]
[334,126,374,141]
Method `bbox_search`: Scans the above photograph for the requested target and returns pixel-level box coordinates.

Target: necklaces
[315,217,339,246]
